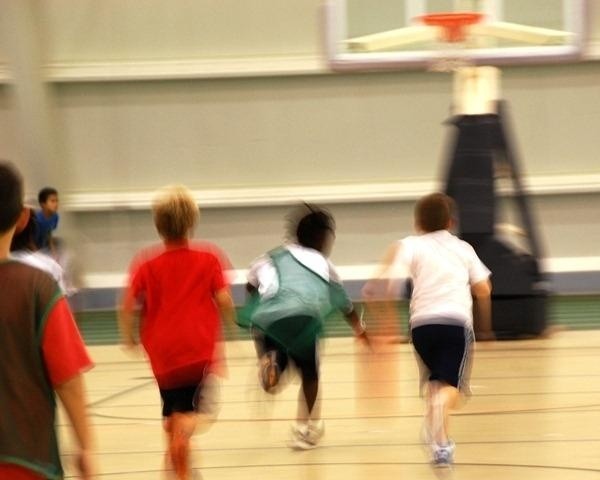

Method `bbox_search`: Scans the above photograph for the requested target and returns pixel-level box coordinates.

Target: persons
[362,191,493,469]
[35,187,60,265]
[1,158,99,480]
[232,200,374,453]
[114,183,240,479]
[9,210,67,301]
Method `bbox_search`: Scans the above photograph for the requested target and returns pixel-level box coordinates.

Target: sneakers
[259,349,280,394]
[432,442,455,464]
[291,420,323,451]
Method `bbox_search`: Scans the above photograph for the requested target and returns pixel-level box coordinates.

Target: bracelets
[351,319,368,339]
